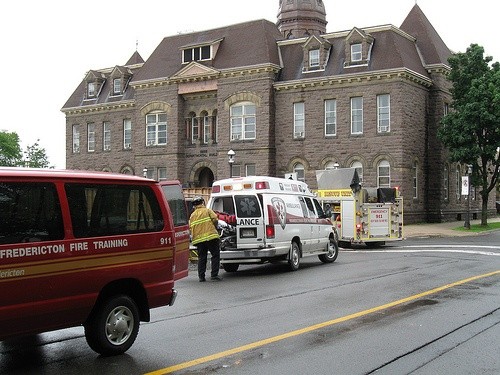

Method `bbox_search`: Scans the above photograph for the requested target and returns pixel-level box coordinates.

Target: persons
[188,194,223,283]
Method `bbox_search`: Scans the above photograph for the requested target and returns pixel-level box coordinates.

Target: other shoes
[199,278,206,282]
[211,275,222,280]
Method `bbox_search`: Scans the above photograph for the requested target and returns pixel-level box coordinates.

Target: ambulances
[206,176,340,274]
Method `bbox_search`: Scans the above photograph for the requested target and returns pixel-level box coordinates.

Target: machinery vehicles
[308,168,404,248]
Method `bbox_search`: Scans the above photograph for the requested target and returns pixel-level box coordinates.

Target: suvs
[0,165,179,358]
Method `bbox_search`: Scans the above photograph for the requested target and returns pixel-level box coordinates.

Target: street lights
[226,147,236,179]
[464,164,472,229]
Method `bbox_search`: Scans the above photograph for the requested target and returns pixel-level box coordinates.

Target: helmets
[191,196,205,206]
